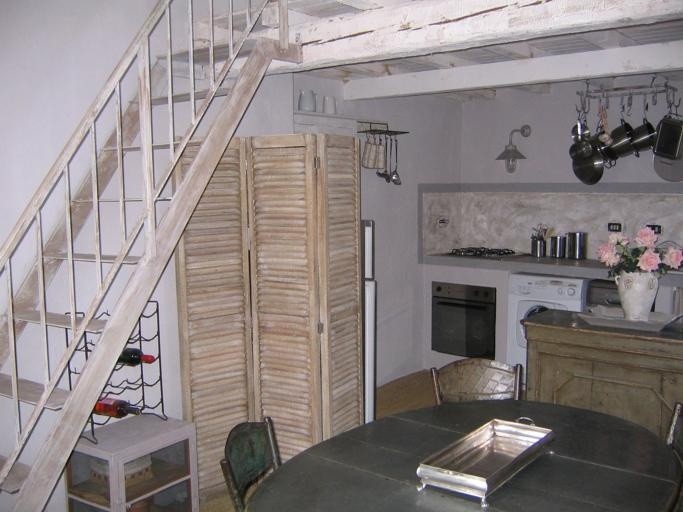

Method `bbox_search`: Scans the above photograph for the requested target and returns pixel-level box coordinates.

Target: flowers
[597,226,683,278]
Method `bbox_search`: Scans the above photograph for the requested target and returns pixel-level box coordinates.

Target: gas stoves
[440,246,529,259]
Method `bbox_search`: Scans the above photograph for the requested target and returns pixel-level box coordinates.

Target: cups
[297,88,316,112]
[322,95,337,114]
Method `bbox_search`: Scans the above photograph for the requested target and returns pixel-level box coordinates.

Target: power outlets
[608,223,623,232]
[646,225,661,234]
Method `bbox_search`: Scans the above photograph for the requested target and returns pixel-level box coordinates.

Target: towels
[674,287,683,315]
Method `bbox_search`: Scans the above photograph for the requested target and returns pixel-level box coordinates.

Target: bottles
[115,347,155,367]
[91,398,140,418]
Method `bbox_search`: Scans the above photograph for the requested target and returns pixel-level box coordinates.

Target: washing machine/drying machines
[507,273,588,394]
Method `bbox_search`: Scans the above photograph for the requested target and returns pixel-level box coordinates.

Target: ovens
[431,280,496,361]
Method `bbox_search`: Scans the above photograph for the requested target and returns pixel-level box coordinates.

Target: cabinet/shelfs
[65,299,168,444]
[63,413,200,511]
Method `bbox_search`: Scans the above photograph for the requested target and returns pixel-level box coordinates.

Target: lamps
[495,124,531,173]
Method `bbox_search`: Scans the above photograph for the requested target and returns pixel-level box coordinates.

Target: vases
[614,270,659,322]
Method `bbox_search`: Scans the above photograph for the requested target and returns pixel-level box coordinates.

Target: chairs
[666,403,682,466]
[431,359,523,405]
[220,417,284,511]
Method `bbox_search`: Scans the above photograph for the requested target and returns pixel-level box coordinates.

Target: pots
[608,117,655,158]
[570,118,616,186]
